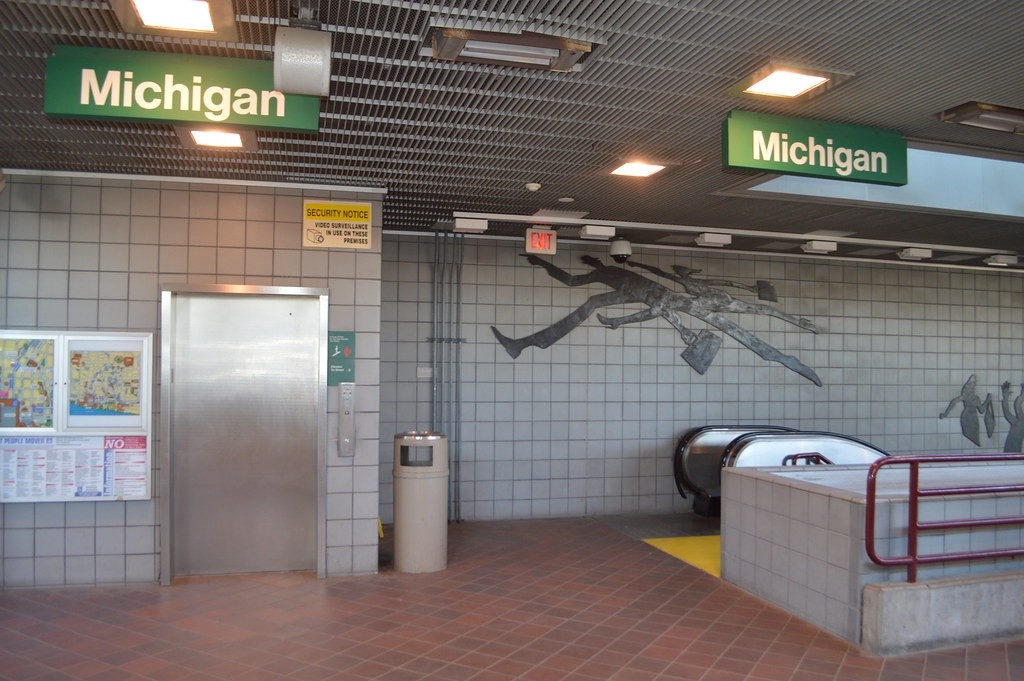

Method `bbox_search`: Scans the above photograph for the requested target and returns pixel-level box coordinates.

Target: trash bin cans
[392,430,450,574]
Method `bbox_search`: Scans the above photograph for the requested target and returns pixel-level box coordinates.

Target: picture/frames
[63,337,148,433]
[0,334,58,434]
